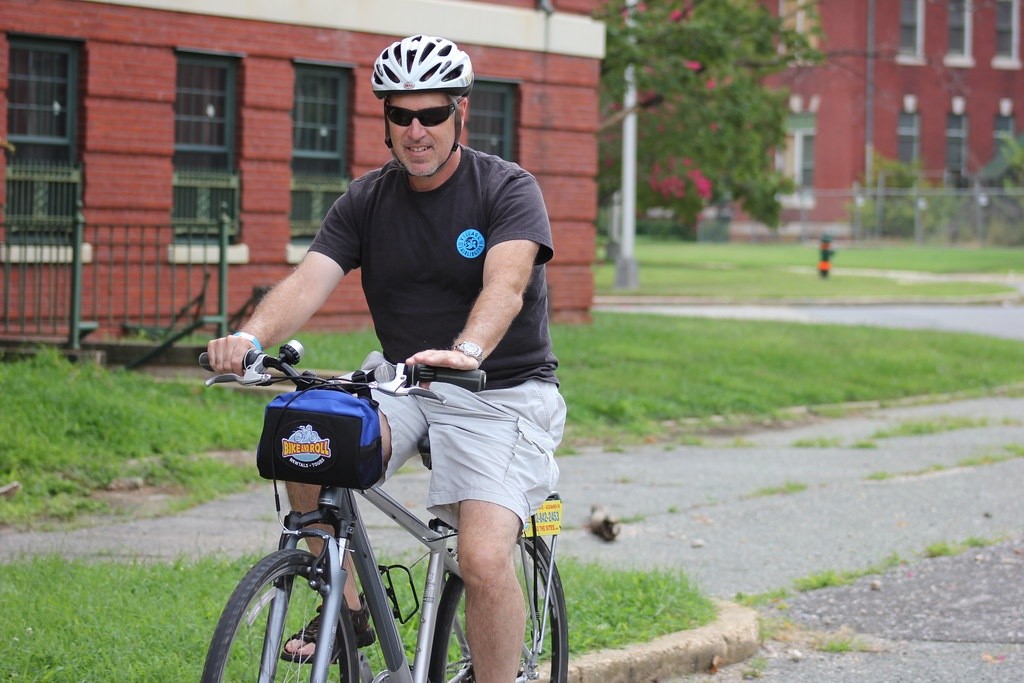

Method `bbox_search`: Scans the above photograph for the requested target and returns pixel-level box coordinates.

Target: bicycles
[198,340,570,683]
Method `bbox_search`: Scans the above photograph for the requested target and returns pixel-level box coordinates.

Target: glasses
[384,97,462,127]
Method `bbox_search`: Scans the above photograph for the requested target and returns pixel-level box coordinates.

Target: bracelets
[233,332,262,351]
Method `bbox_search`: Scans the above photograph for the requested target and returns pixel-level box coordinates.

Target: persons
[205,33,569,683]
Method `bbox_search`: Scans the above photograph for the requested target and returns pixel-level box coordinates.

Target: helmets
[370,34,474,97]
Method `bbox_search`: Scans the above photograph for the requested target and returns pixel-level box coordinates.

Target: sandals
[281,595,376,665]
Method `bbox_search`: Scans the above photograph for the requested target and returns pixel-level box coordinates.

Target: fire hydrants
[817,234,834,277]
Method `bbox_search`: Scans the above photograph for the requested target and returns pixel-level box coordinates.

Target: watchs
[450,343,483,368]
